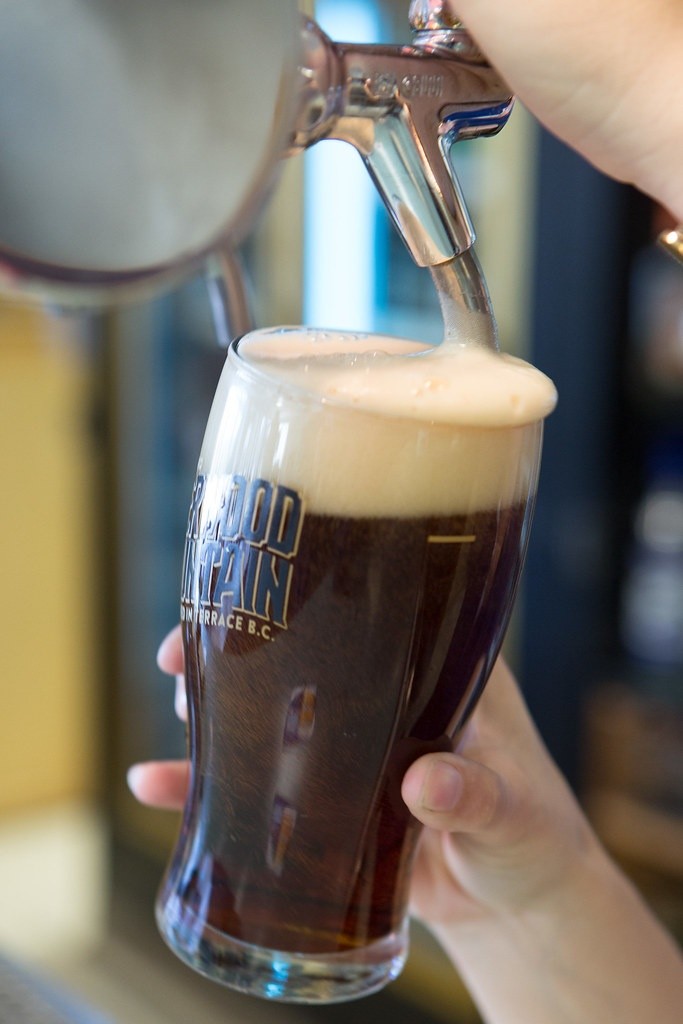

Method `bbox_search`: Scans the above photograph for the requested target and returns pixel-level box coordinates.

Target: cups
[155,324,545,1006]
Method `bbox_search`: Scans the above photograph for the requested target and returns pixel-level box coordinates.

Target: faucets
[330,34,517,274]
